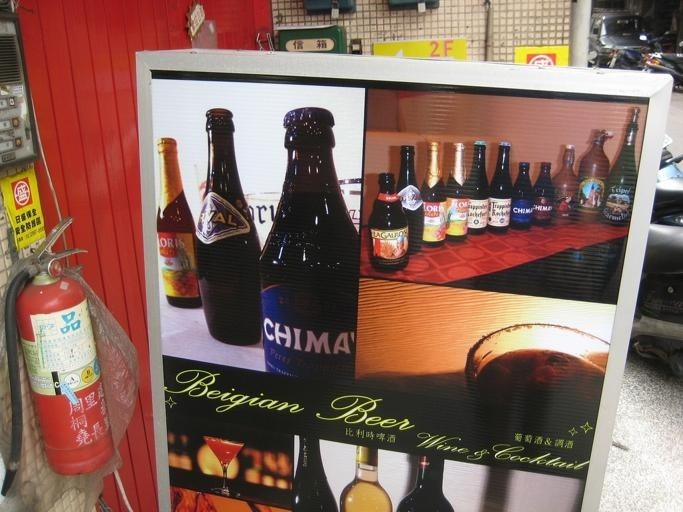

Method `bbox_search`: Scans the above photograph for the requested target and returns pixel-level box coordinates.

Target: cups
[465,322,615,459]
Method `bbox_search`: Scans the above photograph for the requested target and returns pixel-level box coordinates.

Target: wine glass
[205,434,245,497]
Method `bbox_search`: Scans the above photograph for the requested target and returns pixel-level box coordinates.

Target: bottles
[197,108,263,346]
[257,105,360,384]
[398,453,457,511]
[339,443,393,511]
[366,107,641,273]
[292,432,338,512]
[155,136,200,309]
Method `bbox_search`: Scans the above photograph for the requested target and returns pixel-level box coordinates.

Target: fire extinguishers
[1,215,115,497]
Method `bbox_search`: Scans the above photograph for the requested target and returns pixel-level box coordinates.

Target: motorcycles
[629,221,683,376]
[641,40,683,91]
[608,30,662,71]
[650,133,683,225]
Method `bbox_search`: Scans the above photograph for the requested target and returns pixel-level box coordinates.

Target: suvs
[590,10,659,69]
